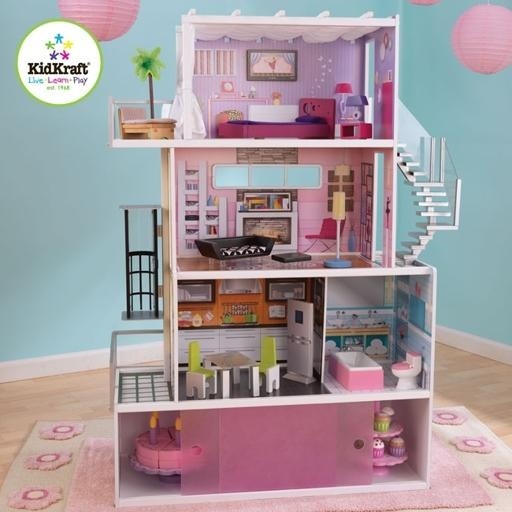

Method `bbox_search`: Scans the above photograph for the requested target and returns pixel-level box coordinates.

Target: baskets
[195,235,276,258]
